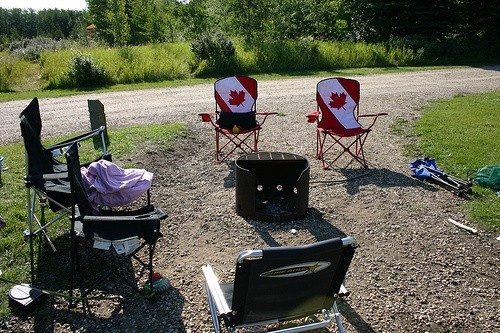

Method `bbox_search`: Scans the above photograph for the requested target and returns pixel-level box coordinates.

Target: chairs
[19,97,169,308]
[198,75,278,161]
[305,77,388,170]
[202,236,357,333]
